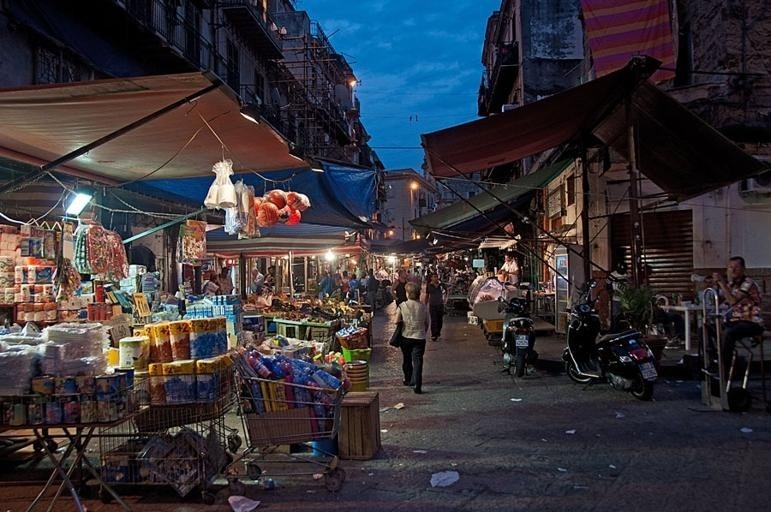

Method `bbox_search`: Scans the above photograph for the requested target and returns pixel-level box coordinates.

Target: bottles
[229,339,344,433]
[672,286,721,310]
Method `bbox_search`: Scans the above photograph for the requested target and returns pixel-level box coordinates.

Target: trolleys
[129,366,243,453]
[97,372,233,506]
[225,351,351,495]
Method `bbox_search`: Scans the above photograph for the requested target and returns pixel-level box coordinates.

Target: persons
[393,281,429,393]
[604,256,764,380]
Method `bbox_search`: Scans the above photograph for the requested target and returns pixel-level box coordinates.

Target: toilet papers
[14,266,57,320]
[119,317,228,402]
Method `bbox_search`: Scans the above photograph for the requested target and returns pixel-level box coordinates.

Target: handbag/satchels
[390,306,404,348]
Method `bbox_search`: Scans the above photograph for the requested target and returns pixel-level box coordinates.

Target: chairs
[622,290,770,375]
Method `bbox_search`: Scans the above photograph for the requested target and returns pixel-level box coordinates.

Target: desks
[0,404,149,512]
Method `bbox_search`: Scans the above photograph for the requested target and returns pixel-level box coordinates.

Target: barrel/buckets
[345,360,369,392]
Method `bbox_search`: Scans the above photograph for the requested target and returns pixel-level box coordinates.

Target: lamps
[286,140,303,161]
[306,156,325,173]
[238,85,262,126]
[60,184,95,217]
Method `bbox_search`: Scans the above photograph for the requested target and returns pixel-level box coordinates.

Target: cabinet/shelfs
[466,273,521,344]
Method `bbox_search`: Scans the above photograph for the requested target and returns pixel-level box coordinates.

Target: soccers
[251,190,310,228]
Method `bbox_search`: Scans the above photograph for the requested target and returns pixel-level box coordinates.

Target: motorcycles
[497,293,555,377]
[562,279,660,401]
